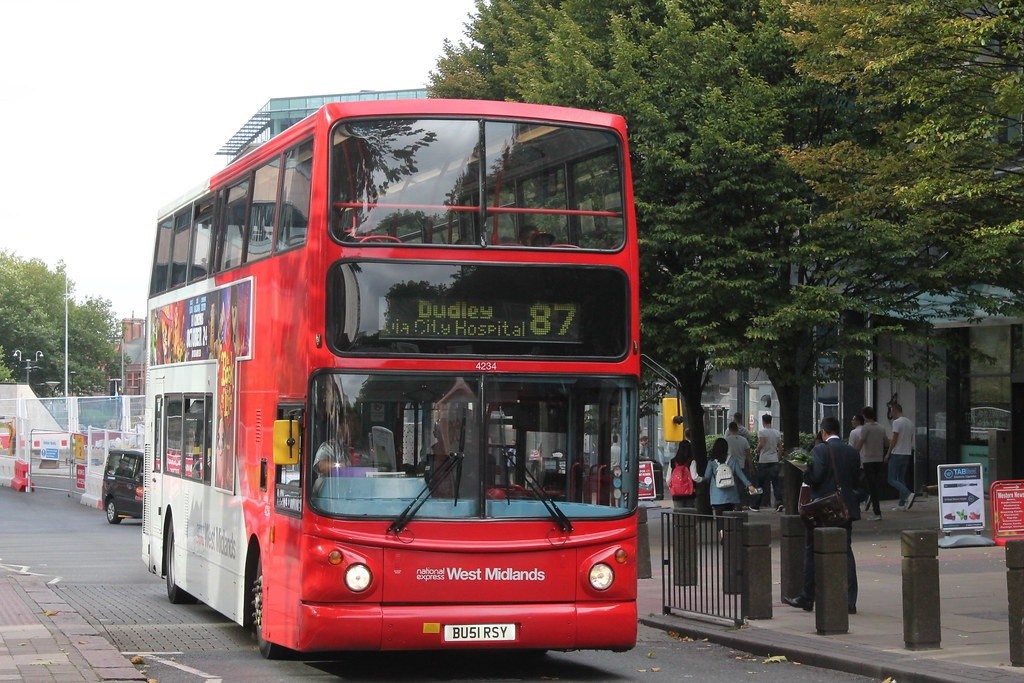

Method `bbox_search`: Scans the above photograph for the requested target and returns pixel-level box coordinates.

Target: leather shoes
[847,605,855,614]
[784,595,813,611]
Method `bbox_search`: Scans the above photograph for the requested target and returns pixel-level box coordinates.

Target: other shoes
[777,505,784,512]
[904,492,915,510]
[893,506,905,511]
[749,505,761,512]
[866,514,883,522]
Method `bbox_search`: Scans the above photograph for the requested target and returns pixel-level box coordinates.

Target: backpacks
[669,460,694,498]
[712,456,734,489]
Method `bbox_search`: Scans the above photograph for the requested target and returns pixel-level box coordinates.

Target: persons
[610,435,621,471]
[725,422,755,511]
[215,414,227,489]
[784,417,861,613]
[750,415,784,512]
[666,440,704,506]
[684,428,690,442]
[639,436,650,460]
[705,437,757,544]
[313,408,369,476]
[724,412,750,443]
[857,407,888,521]
[518,225,539,244]
[847,415,872,511]
[506,439,517,467]
[884,403,916,512]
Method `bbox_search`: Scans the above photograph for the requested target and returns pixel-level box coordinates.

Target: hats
[530,231,555,247]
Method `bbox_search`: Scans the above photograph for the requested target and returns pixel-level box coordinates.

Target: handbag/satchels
[800,493,848,531]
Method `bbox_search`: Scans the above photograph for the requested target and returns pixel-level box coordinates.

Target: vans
[102,450,143,524]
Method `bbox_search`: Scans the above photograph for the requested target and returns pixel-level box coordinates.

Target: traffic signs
[937,463,986,533]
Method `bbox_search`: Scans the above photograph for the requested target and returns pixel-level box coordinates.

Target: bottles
[749,489,763,495]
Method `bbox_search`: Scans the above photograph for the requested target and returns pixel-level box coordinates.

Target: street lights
[46,381,61,417]
[69,370,77,396]
[13,350,44,385]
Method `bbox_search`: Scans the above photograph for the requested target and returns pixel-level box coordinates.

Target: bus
[140,98,685,659]
